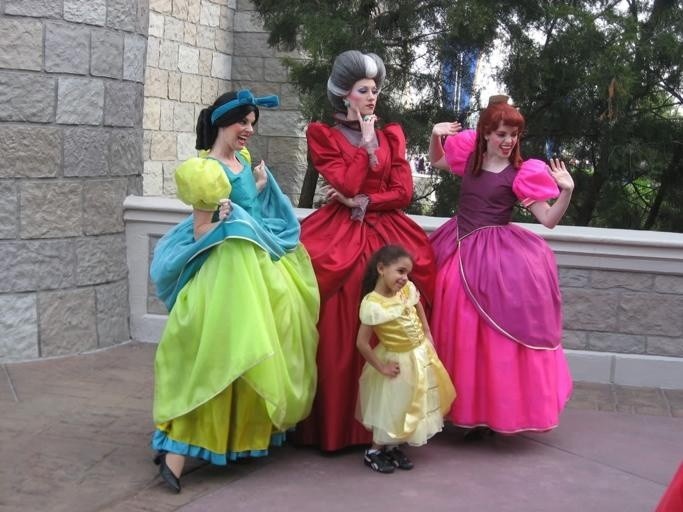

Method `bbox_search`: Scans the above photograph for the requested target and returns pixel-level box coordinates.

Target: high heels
[152,449,183,493]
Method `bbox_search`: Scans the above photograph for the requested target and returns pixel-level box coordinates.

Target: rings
[363,113,370,121]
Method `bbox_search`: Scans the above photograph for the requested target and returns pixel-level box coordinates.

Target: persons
[428,95,575,439]
[352,244,456,474]
[302,51,438,453]
[148,90,321,493]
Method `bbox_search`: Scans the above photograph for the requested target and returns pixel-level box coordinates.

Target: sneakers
[364,449,395,473]
[383,448,414,471]
